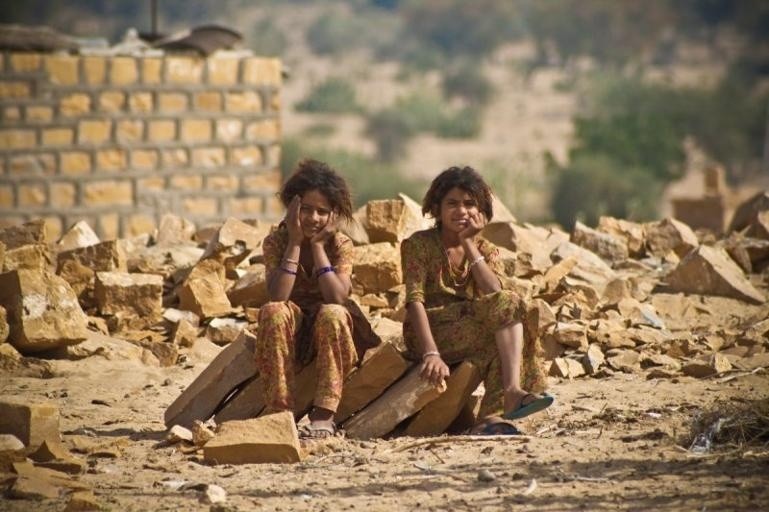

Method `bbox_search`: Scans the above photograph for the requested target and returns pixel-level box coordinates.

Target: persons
[258,160,381,439]
[400,166,554,435]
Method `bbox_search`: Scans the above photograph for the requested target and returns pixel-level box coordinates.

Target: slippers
[500,392,554,420]
[299,424,336,440]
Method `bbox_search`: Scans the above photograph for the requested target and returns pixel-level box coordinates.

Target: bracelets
[316,266,335,278]
[279,258,300,274]
[423,351,441,361]
[470,255,484,266]
